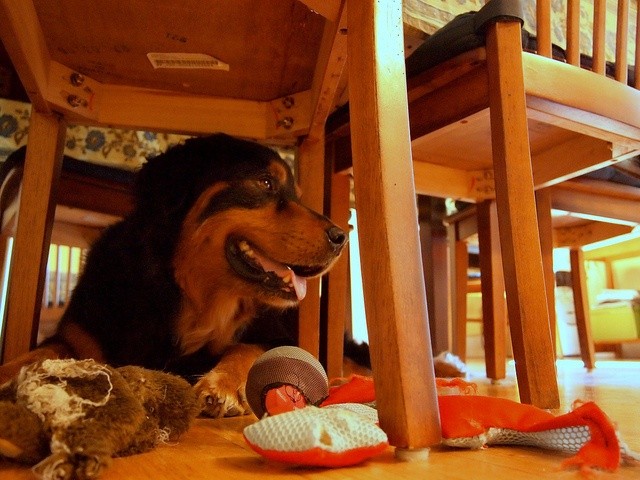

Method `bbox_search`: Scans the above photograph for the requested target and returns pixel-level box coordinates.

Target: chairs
[442,157,639,385]
[324,0,639,417]
[1,0,445,450]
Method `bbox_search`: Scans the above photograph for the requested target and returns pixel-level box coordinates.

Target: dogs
[36,134,349,419]
